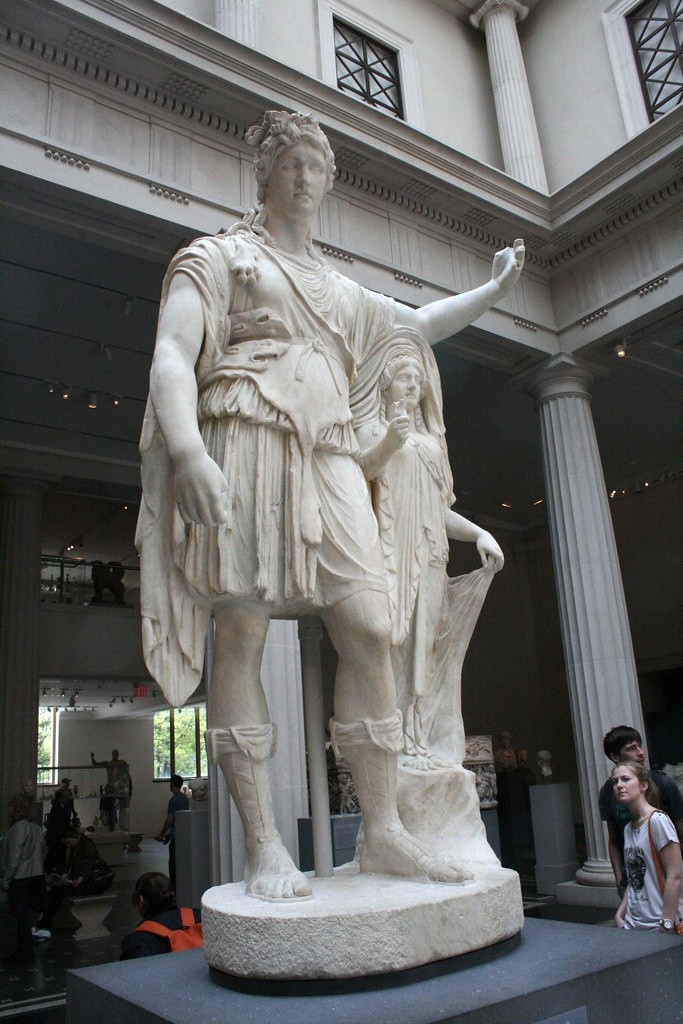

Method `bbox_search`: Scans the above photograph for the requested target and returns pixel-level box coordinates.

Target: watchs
[658,919,675,930]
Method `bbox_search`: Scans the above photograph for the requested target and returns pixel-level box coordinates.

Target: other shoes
[31,927,51,938]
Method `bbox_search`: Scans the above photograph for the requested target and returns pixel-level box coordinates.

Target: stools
[126,832,144,852]
[65,830,128,868]
[62,890,117,942]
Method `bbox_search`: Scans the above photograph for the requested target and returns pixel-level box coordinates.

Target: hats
[62,778,72,783]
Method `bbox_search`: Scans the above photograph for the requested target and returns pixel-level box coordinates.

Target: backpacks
[135,908,205,953]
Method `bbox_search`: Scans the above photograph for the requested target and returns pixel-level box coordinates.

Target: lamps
[88,393,97,409]
[75,691,79,697]
[42,689,46,696]
[110,696,134,707]
[47,705,96,712]
[609,484,641,498]
[61,691,65,697]
[615,336,630,357]
[48,382,54,393]
[644,472,667,486]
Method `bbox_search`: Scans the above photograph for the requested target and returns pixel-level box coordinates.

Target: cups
[74,784,79,795]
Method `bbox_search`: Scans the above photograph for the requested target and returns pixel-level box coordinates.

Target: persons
[612,762,683,935]
[90,749,126,785]
[535,750,554,777]
[118,871,201,962]
[136,110,528,902]
[154,775,189,889]
[598,726,683,900]
[0,778,129,969]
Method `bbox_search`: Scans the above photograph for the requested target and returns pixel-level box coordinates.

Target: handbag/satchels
[85,860,114,890]
